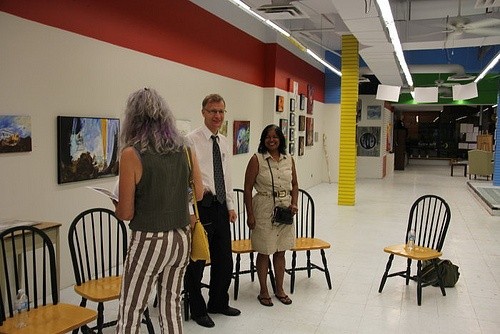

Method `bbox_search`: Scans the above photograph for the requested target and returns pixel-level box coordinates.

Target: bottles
[14,289,29,328]
[407,227,415,249]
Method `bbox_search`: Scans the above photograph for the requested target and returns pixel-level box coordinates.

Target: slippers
[257,294,273,306]
[275,294,292,305]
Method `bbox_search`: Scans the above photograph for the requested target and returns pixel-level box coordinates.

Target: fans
[414,0,500,36]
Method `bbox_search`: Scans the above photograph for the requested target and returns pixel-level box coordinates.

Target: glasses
[204,108,227,114]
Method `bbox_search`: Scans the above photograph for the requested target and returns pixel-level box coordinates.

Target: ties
[211,135,226,204]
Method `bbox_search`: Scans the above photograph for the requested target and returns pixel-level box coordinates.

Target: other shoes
[207,297,240,316]
[190,307,215,327]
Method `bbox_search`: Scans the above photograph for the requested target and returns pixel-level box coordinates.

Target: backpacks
[413,258,460,288]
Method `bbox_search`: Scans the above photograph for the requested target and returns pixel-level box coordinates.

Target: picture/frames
[276,80,314,156]
[233,121,250,155]
[56,116,121,184]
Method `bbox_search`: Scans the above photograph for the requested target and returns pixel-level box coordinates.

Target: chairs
[0,226,97,334]
[284,189,332,294]
[468,149,494,181]
[379,194,451,306]
[68,208,156,334]
[231,189,276,300]
[153,256,210,321]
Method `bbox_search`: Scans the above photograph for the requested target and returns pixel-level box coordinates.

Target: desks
[451,160,469,177]
[0,221,63,307]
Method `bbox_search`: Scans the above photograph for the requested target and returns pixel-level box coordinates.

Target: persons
[244,124,300,306]
[110,87,203,334]
[188,95,242,328]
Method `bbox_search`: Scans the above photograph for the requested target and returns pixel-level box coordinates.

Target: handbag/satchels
[271,206,293,225]
[190,221,211,263]
